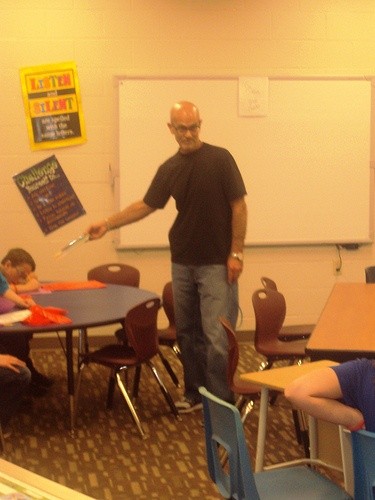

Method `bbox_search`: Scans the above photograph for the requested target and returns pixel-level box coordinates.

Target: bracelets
[351,420,364,431]
[104,218,111,231]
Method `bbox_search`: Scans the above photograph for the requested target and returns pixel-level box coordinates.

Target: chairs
[79,263,180,438]
[200,249,375,500]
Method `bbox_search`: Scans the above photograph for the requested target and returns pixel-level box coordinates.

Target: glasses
[15,267,27,279]
[172,124,200,133]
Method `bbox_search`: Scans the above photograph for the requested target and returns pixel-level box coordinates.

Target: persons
[81,100,247,415]
[284,360,375,434]
[0,248,52,426]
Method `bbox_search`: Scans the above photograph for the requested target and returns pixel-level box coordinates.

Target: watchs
[229,251,244,260]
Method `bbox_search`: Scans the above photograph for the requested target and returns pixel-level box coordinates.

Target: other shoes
[33,371,54,386]
[32,385,47,397]
[175,395,202,409]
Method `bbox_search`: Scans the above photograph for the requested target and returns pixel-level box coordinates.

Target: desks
[303,279,374,364]
[239,359,354,493]
[0,280,161,438]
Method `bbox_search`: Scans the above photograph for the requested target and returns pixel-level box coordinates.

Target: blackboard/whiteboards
[115,73,374,251]
